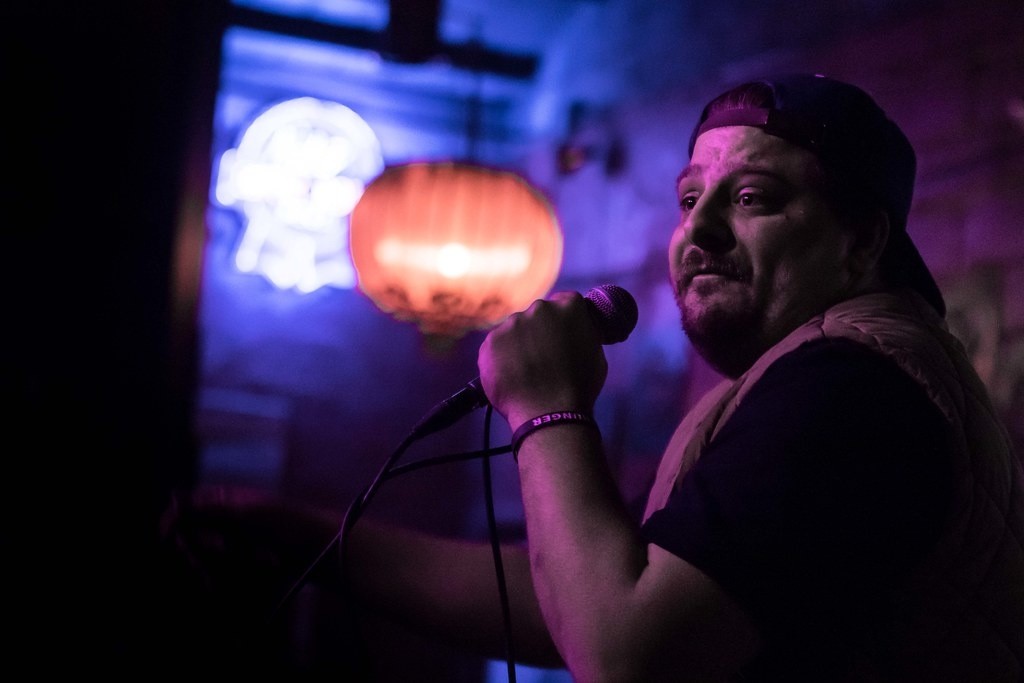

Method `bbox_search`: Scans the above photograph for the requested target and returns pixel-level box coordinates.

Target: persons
[476,75,1024,683]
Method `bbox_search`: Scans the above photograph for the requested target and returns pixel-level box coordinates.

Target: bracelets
[510,411,598,460]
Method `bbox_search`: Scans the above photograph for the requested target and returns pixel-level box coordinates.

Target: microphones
[409,283,639,438]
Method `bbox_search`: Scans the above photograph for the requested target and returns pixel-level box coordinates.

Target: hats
[685,73,946,320]
